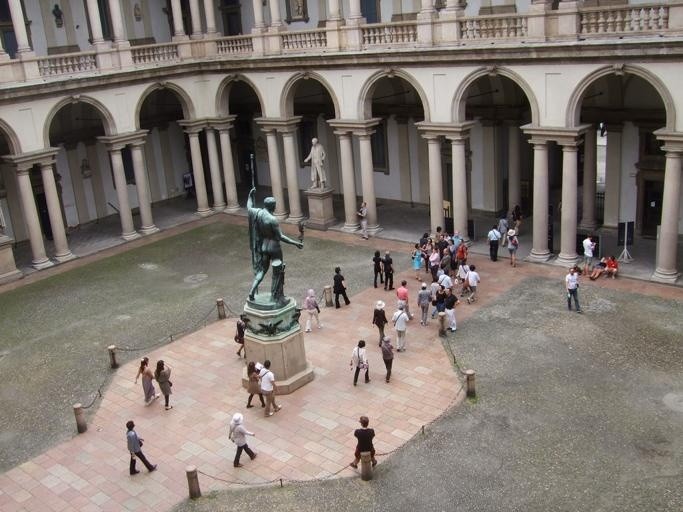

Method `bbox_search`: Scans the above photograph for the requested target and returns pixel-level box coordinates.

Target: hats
[507,229,516,237]
[232,413,242,424]
[375,301,384,309]
[422,282,427,287]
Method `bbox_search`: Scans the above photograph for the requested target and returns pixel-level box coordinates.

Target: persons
[259,359,282,417]
[126,419,157,475]
[134,356,160,407]
[357,201,368,240]
[304,288,322,333]
[154,360,173,411]
[236,314,246,358]
[487,205,524,268]
[350,415,378,468]
[304,137,327,189]
[228,412,257,468]
[246,186,303,301]
[581,232,618,281]
[332,225,480,387]
[565,268,581,313]
[246,361,265,408]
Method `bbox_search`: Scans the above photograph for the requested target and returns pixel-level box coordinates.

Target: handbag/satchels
[358,363,368,369]
[464,280,469,287]
[512,240,517,246]
